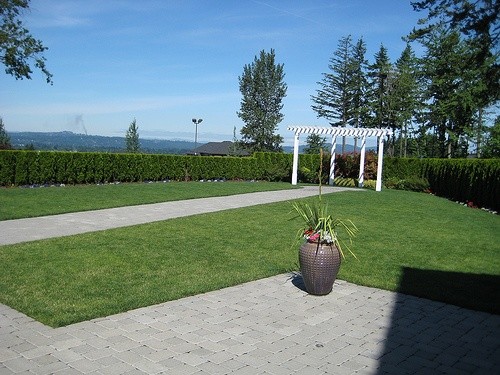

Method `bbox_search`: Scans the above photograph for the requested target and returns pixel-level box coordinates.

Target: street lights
[192,118,202,152]
[379,72,387,129]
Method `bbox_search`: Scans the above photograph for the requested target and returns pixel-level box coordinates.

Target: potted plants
[286,200,360,296]
